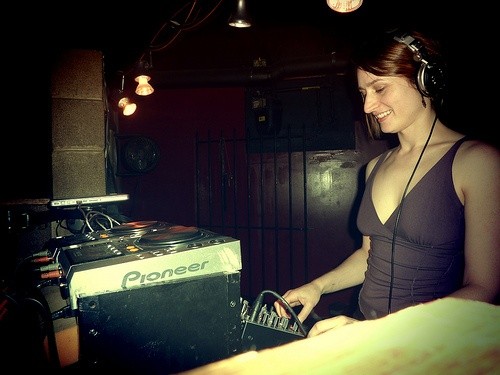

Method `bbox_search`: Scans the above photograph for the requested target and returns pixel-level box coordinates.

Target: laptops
[50,194,128,206]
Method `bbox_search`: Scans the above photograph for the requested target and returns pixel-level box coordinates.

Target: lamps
[325,0,364,13]
[131,69,157,96]
[228,0,256,28]
[107,88,141,116]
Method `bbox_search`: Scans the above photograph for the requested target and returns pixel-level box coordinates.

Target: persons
[274,24,500,337]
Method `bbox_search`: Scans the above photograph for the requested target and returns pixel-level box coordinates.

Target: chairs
[0,290,60,375]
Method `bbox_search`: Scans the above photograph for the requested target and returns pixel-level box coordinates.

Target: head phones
[387,30,446,98]
